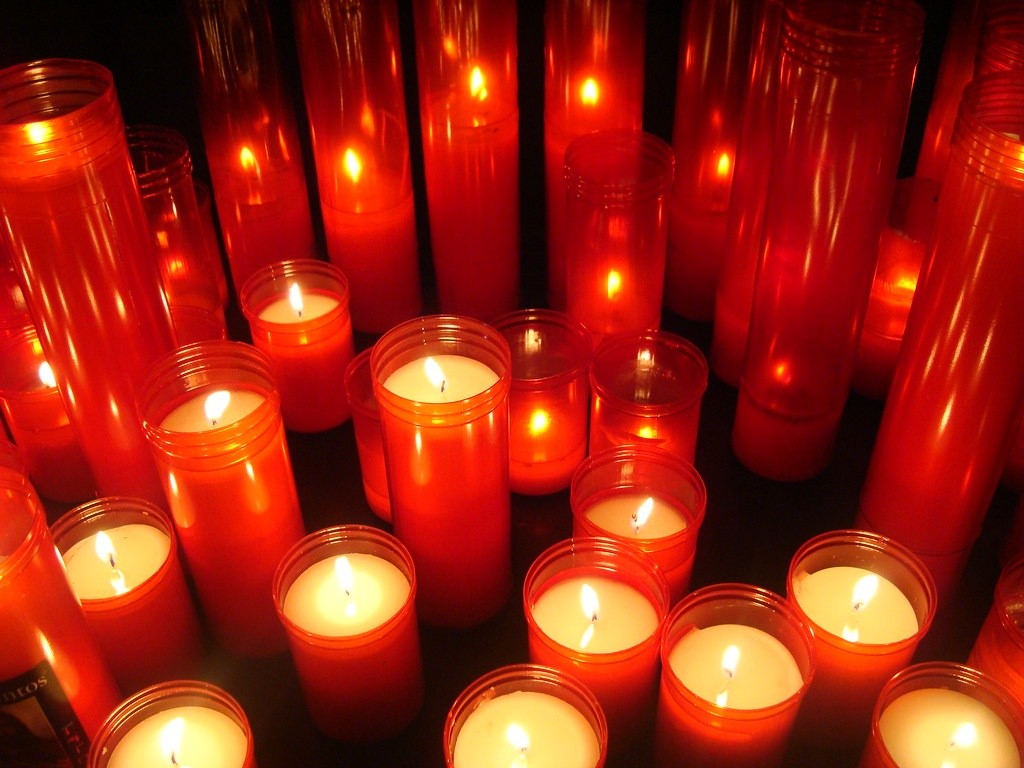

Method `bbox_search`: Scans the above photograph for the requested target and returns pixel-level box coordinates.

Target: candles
[321,143,422,331]
[154,387,303,663]
[253,280,354,431]
[562,238,662,337]
[103,703,248,768]
[59,520,212,695]
[657,624,802,768]
[588,488,691,605]
[860,228,957,335]
[879,685,1020,768]
[282,552,421,741]
[730,353,842,475]
[528,574,661,758]
[0,117,212,561]
[674,141,744,318]
[383,355,514,621]
[452,690,599,767]
[424,62,522,317]
[551,73,645,316]
[9,359,116,506]
[510,407,585,494]
[796,565,918,752]
